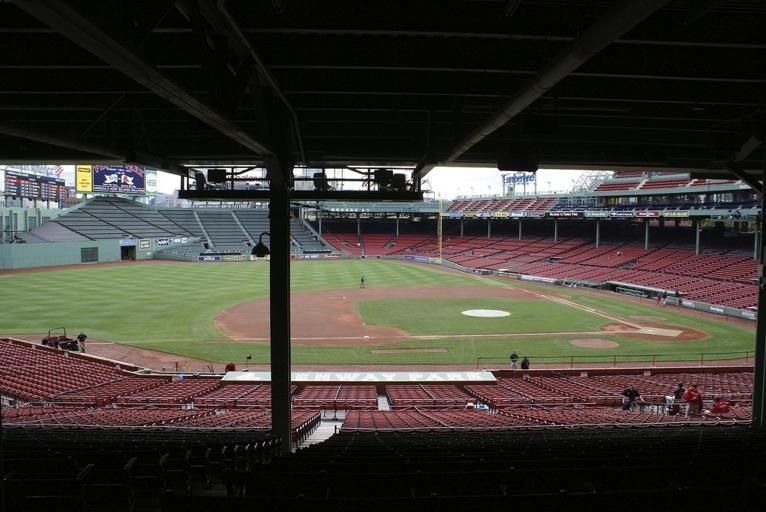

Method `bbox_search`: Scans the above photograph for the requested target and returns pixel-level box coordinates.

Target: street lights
[487,184,492,195]
[546,180,551,192]
[469,183,474,194]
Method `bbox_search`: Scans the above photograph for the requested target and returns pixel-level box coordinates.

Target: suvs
[41,325,79,353]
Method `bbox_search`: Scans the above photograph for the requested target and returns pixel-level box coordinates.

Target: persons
[77,332,87,353]
[521,356,529,369]
[510,351,518,369]
[360,273,365,288]
[668,381,729,416]
[620,383,640,411]
[617,249,623,256]
[225,360,235,372]
[656,288,681,307]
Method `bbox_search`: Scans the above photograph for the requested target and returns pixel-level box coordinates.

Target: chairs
[319,339,764,511]
[0,338,319,511]
